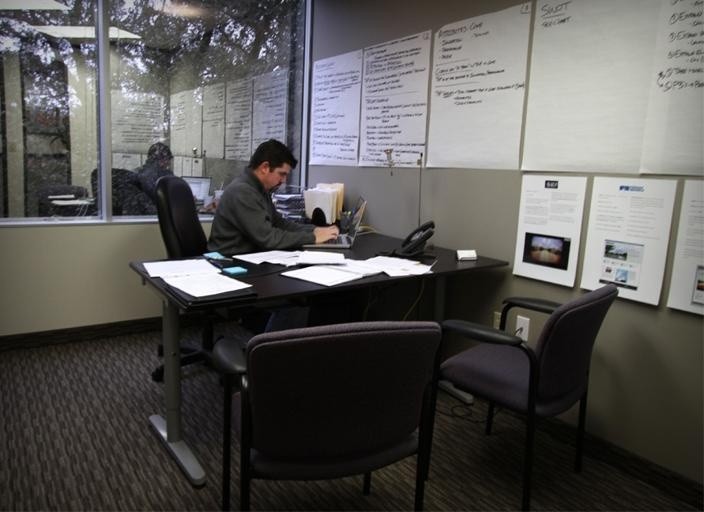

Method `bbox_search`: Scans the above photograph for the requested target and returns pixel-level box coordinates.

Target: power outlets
[492,311,501,330]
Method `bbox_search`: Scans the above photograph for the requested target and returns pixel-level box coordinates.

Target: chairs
[209,317,443,512]
[91,166,157,217]
[33,183,89,218]
[420,282,619,512]
[146,175,240,381]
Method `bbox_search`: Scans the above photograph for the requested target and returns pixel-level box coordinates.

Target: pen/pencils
[209,259,223,268]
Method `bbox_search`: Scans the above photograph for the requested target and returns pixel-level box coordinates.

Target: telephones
[401,220,435,253]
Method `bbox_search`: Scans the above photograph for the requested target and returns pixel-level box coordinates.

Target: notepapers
[222,266,248,275]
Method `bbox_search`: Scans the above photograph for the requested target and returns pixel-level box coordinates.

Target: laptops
[301,195,367,249]
[179,174,212,206]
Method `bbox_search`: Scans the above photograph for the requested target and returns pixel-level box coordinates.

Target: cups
[203,195,213,208]
[339,210,351,231]
[214,189,224,200]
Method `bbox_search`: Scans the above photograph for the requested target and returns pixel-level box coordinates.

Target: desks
[127,233,511,487]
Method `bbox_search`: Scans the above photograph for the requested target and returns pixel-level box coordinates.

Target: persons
[130,142,198,205]
[206,139,340,333]
